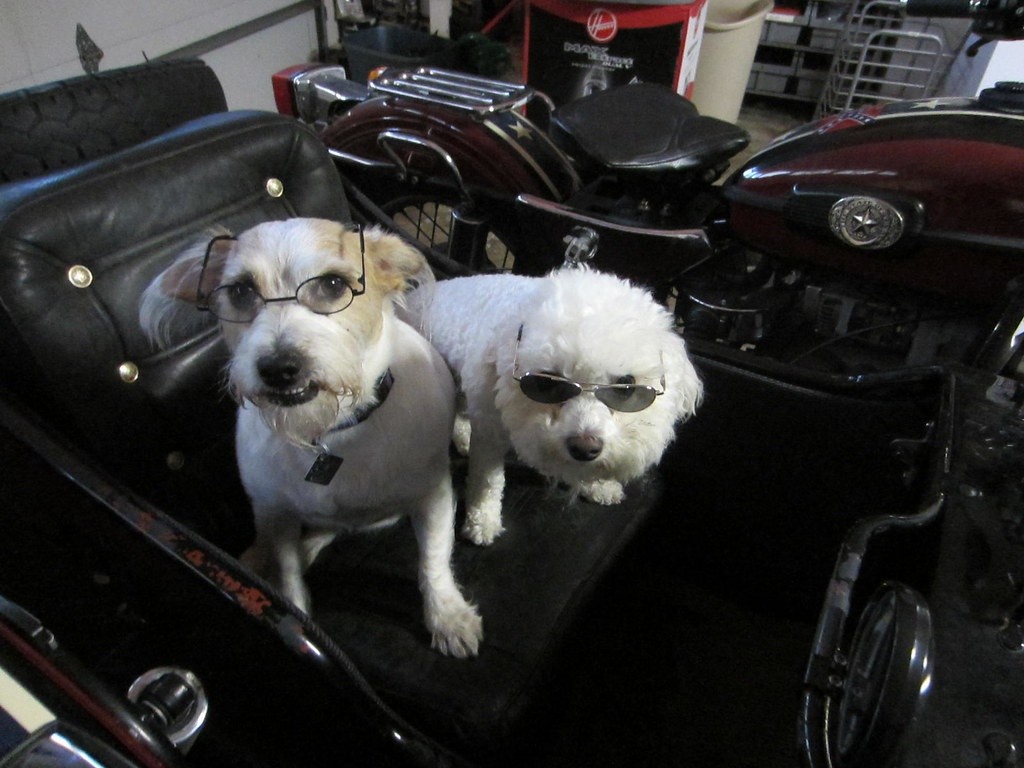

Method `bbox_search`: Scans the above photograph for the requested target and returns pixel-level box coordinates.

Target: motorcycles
[0,57,1023,765]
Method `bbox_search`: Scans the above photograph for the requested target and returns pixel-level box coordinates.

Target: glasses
[513,323,666,412]
[195,221,365,323]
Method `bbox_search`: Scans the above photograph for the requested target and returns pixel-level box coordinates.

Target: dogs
[392,263,708,547]
[134,215,479,660]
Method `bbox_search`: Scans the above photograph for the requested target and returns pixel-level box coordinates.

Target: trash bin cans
[341,22,456,87]
